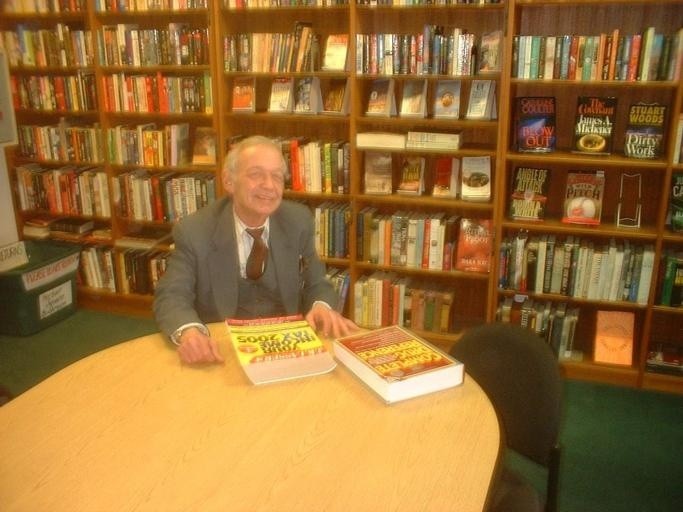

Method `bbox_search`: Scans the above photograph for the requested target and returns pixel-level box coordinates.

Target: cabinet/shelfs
[491,0,683,396]
[1,0,220,320]
[212,0,509,353]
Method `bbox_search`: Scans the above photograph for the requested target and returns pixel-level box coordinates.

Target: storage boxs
[1,240,81,336]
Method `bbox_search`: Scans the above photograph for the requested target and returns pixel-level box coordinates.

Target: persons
[152,136,359,366]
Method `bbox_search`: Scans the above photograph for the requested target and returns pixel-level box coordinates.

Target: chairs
[448,322,563,512]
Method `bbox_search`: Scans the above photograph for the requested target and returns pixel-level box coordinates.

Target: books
[1,1,215,297]
[562,170,604,226]
[511,28,677,81]
[497,230,655,306]
[571,97,617,154]
[623,101,669,160]
[227,314,338,387]
[666,112,683,232]
[333,324,464,404]
[495,297,579,358]
[644,249,683,376]
[220,1,350,315]
[593,309,634,368]
[513,98,557,153]
[353,0,506,335]
[508,167,549,222]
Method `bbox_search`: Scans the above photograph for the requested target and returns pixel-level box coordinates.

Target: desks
[0,317,501,512]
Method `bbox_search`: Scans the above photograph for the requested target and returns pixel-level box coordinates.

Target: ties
[242,226,269,280]
